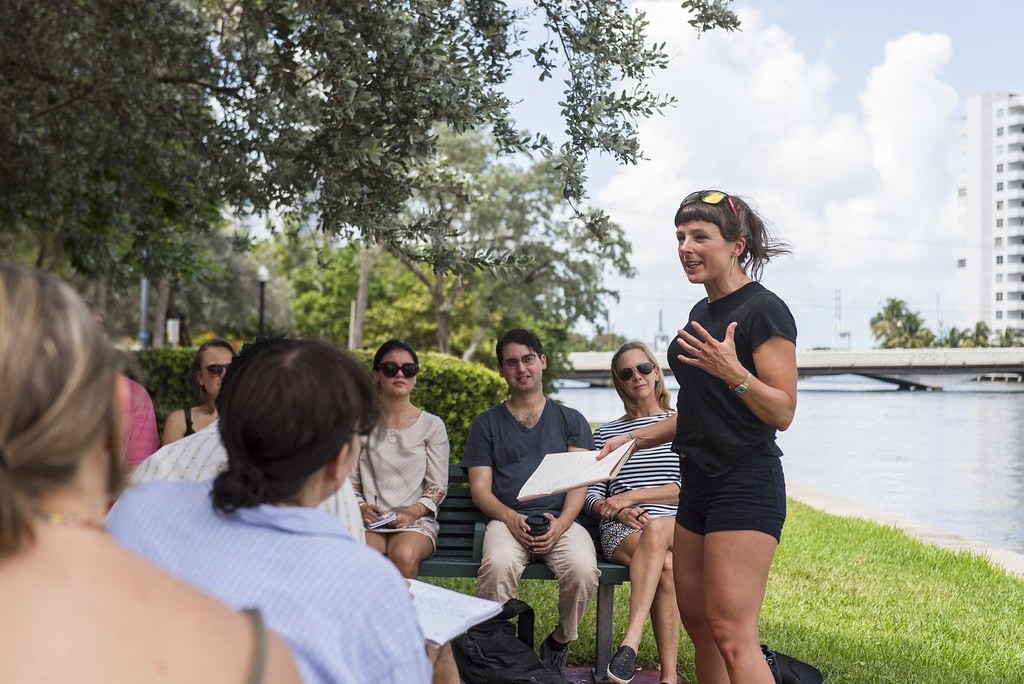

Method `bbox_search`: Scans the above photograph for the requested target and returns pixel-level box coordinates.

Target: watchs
[730,371,750,396]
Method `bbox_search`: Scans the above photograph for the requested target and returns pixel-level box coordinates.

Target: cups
[524,515,550,564]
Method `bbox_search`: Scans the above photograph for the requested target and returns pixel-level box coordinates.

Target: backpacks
[451,597,573,684]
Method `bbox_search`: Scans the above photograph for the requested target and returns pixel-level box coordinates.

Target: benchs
[413,465,630,681]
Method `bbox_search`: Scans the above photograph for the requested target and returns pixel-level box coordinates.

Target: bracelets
[360,502,366,506]
[628,432,639,451]
[416,503,424,514]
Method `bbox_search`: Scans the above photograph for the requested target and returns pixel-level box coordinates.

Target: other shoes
[539,629,569,676]
[606,645,638,684]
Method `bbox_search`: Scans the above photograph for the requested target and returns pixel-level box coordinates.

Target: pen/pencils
[375,496,377,505]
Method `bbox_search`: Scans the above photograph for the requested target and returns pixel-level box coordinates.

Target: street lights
[255,264,269,340]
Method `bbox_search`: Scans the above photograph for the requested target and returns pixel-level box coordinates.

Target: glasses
[200,363,232,375]
[679,190,739,218]
[377,361,419,379]
[502,352,543,369]
[616,362,655,381]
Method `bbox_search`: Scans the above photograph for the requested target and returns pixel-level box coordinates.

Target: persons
[596,188,800,684]
[459,330,599,674]
[104,329,462,684]
[102,367,158,510]
[348,336,451,581]
[162,339,237,446]
[586,337,685,684]
[127,411,363,549]
[0,258,304,684]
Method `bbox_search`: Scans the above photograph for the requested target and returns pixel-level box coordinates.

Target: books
[516,438,635,501]
[404,576,503,649]
[365,511,396,530]
